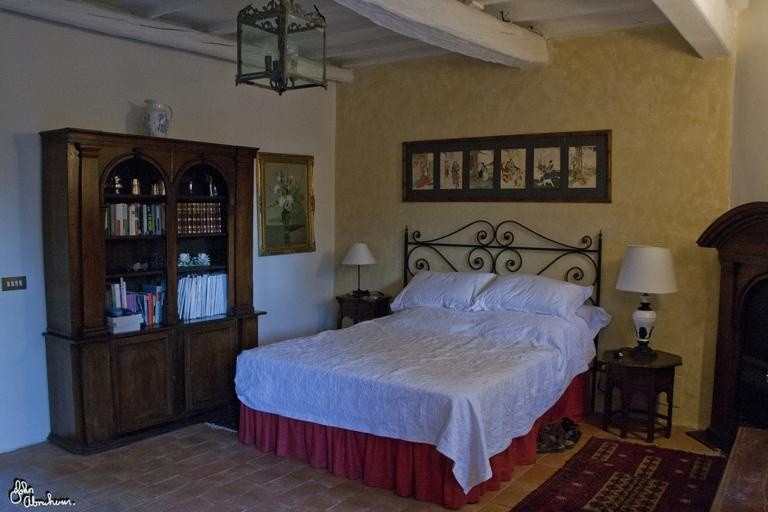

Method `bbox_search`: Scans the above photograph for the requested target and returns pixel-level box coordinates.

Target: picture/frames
[256,152,316,258]
[402,129,613,203]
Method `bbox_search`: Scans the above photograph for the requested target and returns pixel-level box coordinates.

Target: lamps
[616,244,678,363]
[235,0,327,97]
[341,240,377,298]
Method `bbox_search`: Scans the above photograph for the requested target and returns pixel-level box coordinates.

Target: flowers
[336,294,392,330]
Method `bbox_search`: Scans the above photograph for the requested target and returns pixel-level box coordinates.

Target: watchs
[612,350,625,361]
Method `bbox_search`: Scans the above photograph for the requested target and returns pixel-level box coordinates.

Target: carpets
[201,402,239,433]
[509,436,727,512]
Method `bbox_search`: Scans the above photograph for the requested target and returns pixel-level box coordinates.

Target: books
[174,270,231,321]
[176,201,225,235]
[107,277,166,336]
[102,202,168,237]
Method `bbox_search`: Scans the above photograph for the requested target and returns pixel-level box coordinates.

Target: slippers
[562,438,574,449]
[536,429,565,453]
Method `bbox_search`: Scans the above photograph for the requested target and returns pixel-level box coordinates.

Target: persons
[454,159,462,187]
[547,159,556,179]
[442,159,450,185]
[478,162,490,184]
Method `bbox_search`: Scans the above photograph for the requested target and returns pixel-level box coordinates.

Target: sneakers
[548,428,578,443]
[550,417,582,437]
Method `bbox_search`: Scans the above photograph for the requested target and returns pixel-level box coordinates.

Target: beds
[235,219,612,510]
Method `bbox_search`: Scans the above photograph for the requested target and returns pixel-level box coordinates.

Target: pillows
[471,273,594,321]
[391,270,497,312]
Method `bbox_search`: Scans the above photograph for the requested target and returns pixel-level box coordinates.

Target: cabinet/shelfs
[39,127,260,455]
